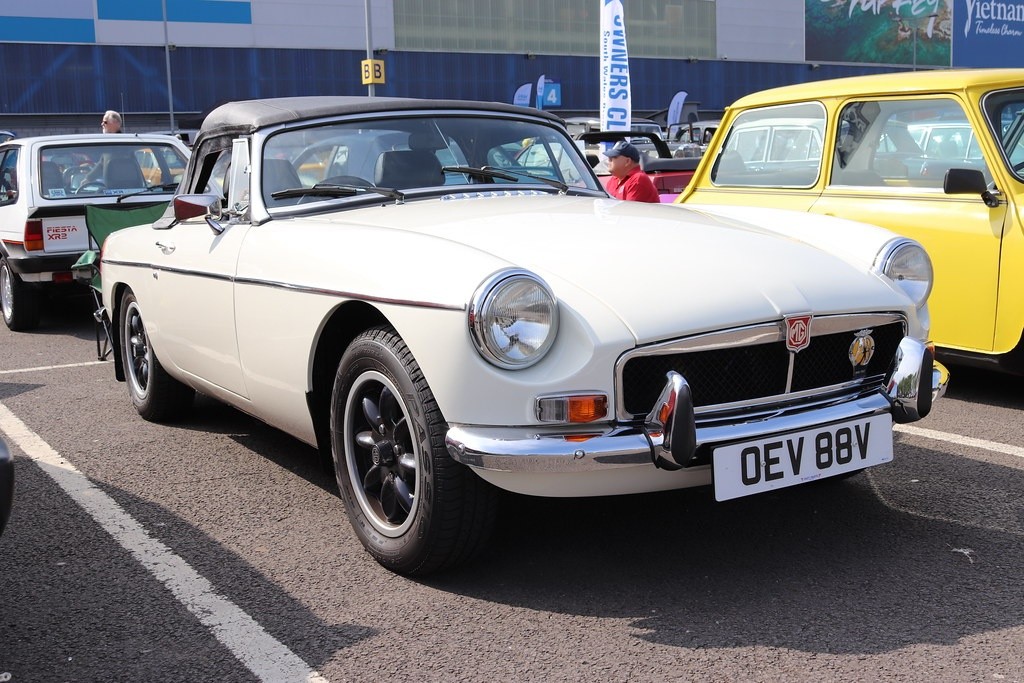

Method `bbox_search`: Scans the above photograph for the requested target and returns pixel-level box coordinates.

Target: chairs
[374,150,446,189]
[104,160,141,190]
[223,158,302,206]
[40,161,63,196]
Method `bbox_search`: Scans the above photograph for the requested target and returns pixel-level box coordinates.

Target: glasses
[101,121,108,124]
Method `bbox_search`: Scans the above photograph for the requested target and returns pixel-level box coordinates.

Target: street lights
[893,14,939,71]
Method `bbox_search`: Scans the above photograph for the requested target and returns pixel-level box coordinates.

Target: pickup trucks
[509,117,699,204]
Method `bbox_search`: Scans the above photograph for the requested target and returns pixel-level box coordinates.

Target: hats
[602,141,639,164]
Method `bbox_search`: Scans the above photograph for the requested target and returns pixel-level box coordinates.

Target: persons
[174,134,188,146]
[77,110,133,185]
[706,131,712,141]
[0,169,17,201]
[604,140,661,203]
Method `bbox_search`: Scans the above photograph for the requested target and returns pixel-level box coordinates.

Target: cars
[0,134,226,333]
[101,95,950,577]
[674,66,1024,390]
[133,147,196,187]
